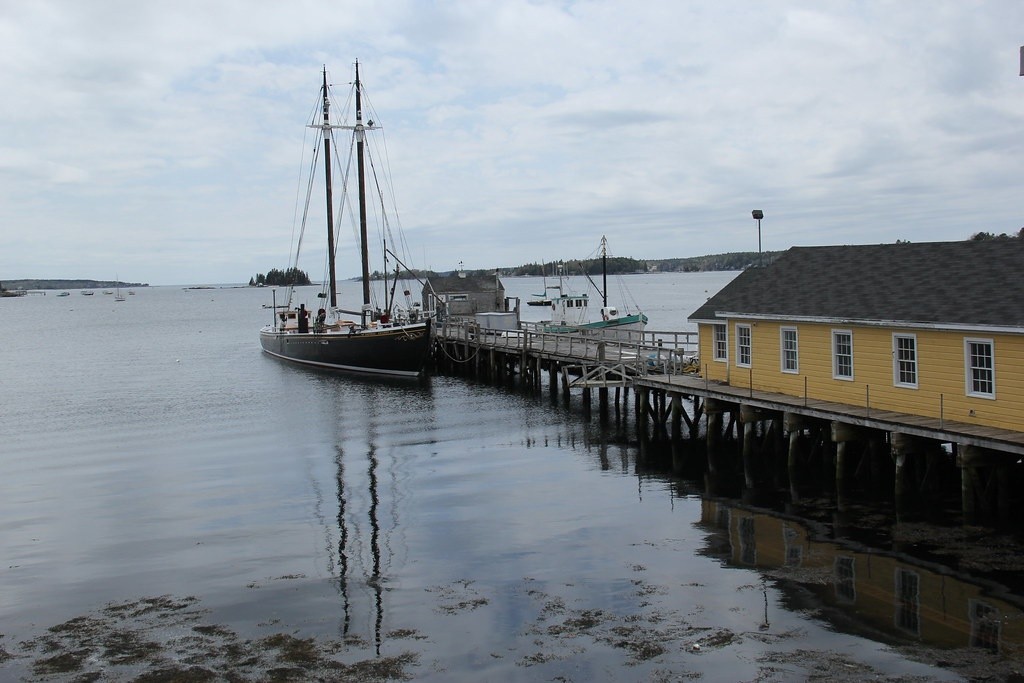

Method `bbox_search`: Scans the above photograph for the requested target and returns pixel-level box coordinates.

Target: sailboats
[259,58,445,378]
[114,274,126,302]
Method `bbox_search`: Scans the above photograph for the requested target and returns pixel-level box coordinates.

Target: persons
[280,319,286,332]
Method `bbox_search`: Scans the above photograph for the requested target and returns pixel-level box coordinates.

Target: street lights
[751,209,765,261]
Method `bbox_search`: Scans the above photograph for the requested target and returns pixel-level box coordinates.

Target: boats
[521,233,649,344]
